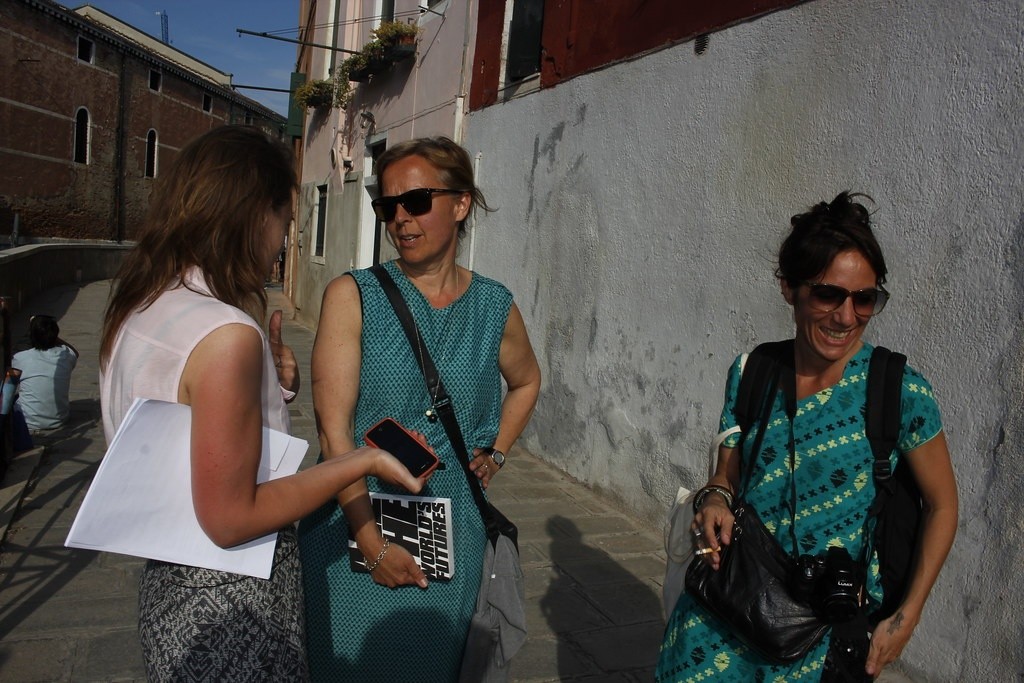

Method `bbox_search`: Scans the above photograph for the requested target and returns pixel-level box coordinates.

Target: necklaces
[406,264,459,423]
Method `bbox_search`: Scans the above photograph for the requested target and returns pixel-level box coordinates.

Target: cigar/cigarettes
[695,546,721,555]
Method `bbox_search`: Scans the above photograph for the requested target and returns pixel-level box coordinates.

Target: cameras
[795,546,864,624]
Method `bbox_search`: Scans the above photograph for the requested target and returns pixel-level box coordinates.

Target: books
[348,491,455,580]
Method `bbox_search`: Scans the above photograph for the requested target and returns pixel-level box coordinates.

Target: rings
[691,528,701,542]
[276,356,281,368]
[482,464,488,468]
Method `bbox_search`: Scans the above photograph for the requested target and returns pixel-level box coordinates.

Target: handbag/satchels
[485,501,520,557]
[685,498,834,665]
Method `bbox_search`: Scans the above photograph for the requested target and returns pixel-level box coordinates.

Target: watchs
[485,447,506,469]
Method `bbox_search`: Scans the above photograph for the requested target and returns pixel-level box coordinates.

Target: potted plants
[294,17,425,116]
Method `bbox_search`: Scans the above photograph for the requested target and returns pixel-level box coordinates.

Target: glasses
[371,188,463,222]
[804,280,890,317]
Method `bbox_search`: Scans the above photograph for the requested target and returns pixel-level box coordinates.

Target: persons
[654,187,959,683]
[99,125,434,683]
[11,315,79,431]
[296,136,541,683]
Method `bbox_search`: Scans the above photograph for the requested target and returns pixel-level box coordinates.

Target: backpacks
[734,339,921,624]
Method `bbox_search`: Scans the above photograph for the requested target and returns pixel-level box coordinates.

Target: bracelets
[364,536,389,571]
[693,485,734,515]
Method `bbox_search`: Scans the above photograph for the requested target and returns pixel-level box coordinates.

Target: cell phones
[364,417,438,479]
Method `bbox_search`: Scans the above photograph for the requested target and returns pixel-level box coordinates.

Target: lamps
[359,111,375,129]
